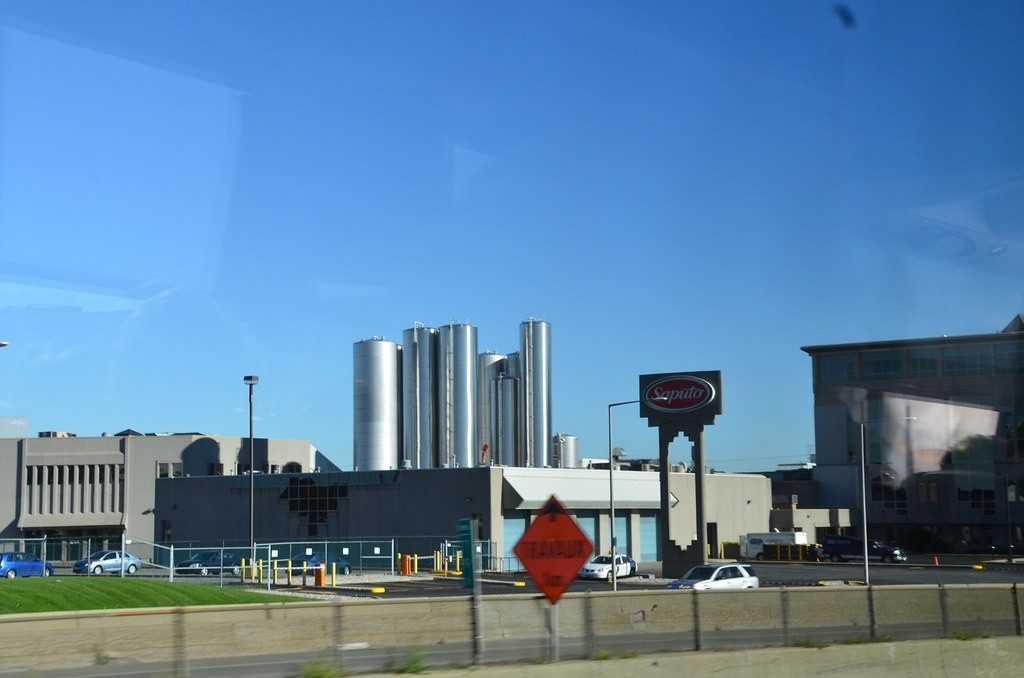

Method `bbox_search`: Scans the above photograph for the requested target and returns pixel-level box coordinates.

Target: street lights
[608,397,667,592]
[860,416,919,586]
[243,375,258,560]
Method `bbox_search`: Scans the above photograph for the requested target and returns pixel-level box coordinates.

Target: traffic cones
[933,555,940,566]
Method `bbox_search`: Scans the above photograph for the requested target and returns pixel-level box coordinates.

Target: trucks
[745,531,808,562]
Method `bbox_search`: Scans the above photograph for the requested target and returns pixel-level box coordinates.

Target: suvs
[822,533,907,564]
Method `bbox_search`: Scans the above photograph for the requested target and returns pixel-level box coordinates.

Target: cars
[285,551,352,576]
[662,562,759,591]
[580,549,638,581]
[72,550,142,575]
[0,551,54,579]
[175,550,241,577]
